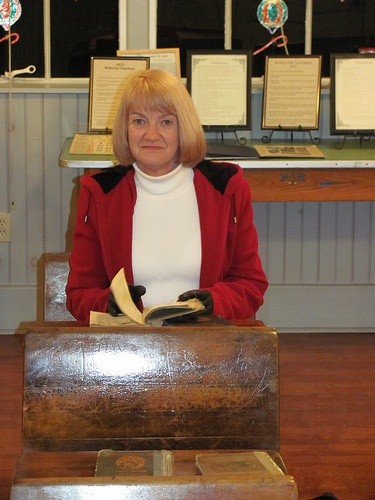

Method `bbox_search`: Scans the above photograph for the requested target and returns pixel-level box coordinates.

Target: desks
[0,331,375,499]
[59,135,375,201]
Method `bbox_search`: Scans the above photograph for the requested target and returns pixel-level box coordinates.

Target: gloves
[176,289,214,316]
[108,284,146,317]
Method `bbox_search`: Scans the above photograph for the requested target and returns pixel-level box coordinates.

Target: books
[89,267,205,329]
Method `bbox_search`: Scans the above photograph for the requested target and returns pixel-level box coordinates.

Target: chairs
[38,253,74,329]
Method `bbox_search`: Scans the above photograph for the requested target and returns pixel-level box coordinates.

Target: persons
[66,69,269,324]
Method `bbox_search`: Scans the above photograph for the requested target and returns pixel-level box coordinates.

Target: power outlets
[0,212,11,241]
